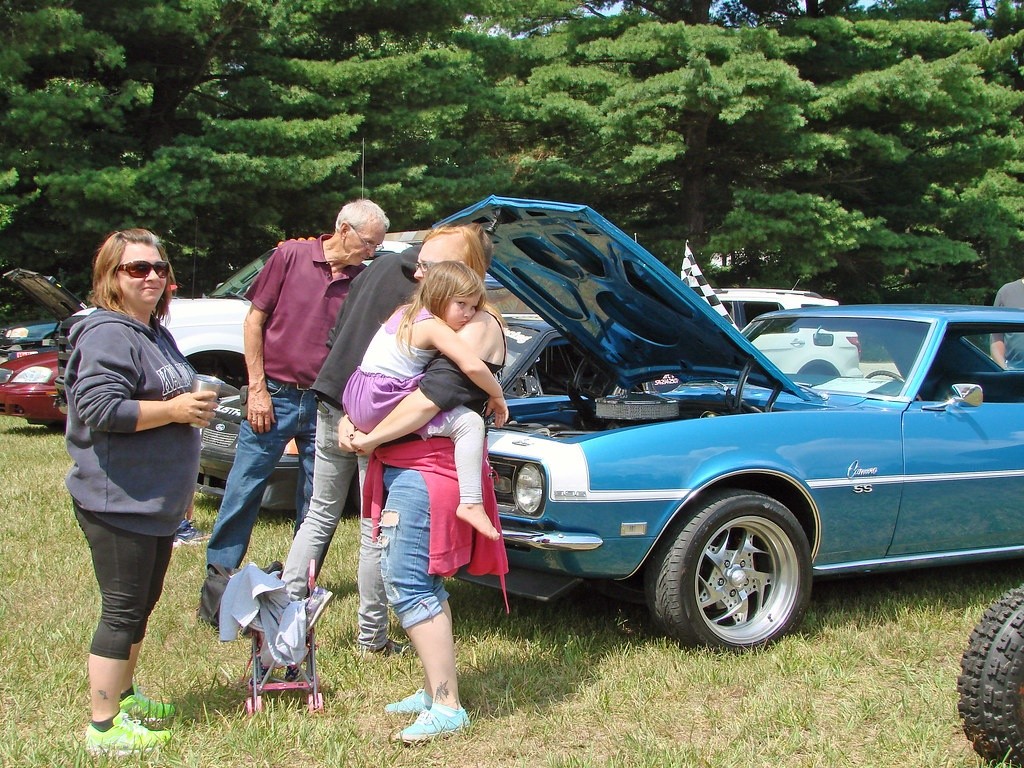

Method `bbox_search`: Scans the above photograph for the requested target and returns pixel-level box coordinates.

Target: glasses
[349,224,384,252]
[113,260,170,278]
[414,262,431,272]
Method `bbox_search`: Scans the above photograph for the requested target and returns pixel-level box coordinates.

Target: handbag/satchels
[197,560,284,629]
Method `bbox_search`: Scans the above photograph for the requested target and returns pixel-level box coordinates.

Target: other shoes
[361,638,403,659]
[304,586,334,633]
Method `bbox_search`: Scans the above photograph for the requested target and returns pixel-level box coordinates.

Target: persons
[64,228,218,758]
[205,197,391,567]
[283,222,507,744]
[987,276,1023,372]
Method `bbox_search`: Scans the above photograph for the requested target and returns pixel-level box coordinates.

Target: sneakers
[384,688,433,714]
[173,519,213,548]
[118,681,176,721]
[396,708,471,743]
[85,709,171,756]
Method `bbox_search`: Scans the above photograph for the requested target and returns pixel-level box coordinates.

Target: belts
[277,381,311,390]
[316,398,329,414]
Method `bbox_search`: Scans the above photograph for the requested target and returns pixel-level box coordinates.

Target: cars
[0,350,72,432]
[0,266,88,354]
[419,193,1024,654]
[194,318,578,512]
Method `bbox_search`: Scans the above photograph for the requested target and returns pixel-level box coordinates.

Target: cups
[188,374,222,429]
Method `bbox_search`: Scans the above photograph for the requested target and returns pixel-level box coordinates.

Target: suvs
[53,240,413,416]
[711,284,863,388]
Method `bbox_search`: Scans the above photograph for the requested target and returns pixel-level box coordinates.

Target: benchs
[936,371,1024,404]
